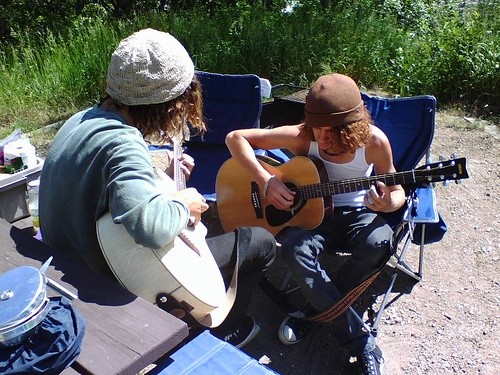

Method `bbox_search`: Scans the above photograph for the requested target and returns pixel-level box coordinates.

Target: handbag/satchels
[0,297,85,375]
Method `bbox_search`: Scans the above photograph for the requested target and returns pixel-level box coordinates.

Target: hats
[105,28,193,105]
[305,73,364,127]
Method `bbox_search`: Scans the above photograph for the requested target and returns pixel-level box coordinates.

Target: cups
[28,180,41,232]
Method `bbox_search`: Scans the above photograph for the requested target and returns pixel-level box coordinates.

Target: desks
[0,218,189,375]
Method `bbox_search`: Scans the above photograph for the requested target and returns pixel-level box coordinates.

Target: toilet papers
[4,141,37,168]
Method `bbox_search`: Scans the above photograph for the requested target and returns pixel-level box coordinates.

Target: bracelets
[263,176,274,198]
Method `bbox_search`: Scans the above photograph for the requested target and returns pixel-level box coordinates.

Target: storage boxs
[0,155,44,223]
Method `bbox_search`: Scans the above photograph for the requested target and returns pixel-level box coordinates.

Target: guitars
[215,153,470,240]
[96,134,226,321]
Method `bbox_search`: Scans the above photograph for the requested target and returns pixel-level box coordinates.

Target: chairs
[143,330,284,375]
[147,71,293,214]
[325,91,446,336]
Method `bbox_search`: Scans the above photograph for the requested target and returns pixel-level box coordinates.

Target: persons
[225,75,406,375]
[38,27,292,349]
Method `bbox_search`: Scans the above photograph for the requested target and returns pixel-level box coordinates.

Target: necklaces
[322,149,346,156]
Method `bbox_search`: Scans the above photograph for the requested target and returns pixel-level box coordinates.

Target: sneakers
[212,317,258,348]
[279,305,324,346]
[357,344,384,375]
